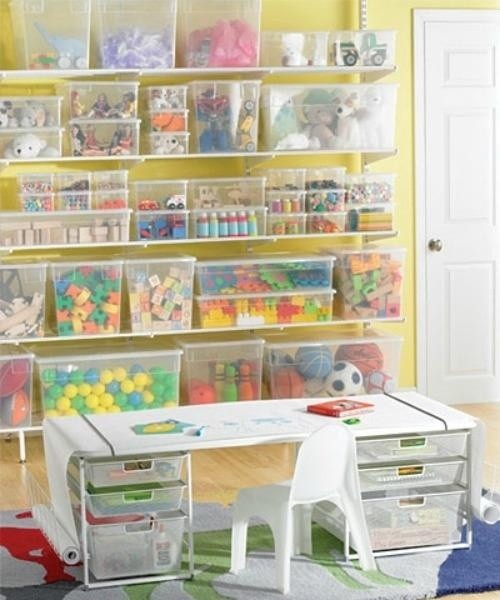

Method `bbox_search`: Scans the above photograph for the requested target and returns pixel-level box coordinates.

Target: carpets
[0,476,500,600]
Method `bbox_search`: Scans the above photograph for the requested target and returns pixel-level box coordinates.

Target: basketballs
[267,367,304,398]
[295,346,334,378]
[0,389,29,426]
[336,344,383,376]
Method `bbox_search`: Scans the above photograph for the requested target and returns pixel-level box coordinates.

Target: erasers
[344,418,359,426]
[196,425,205,437]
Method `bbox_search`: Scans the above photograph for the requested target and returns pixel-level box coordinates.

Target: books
[307,399,375,419]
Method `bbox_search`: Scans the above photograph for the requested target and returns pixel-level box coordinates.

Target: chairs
[229,422,375,594]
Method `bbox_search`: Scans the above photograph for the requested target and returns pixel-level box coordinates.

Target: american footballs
[0,358,29,398]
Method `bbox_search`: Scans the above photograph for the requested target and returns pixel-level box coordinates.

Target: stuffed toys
[271,92,391,151]
[0,100,59,159]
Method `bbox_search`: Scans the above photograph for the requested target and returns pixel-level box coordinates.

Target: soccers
[325,360,363,397]
[261,351,293,383]
[365,367,397,394]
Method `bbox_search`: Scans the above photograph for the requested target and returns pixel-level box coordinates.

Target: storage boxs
[259,83,401,151]
[43,253,126,337]
[251,330,403,401]
[320,245,402,320]
[92,2,178,70]
[0,95,65,129]
[2,344,36,428]
[139,109,190,132]
[1,167,397,248]
[311,431,471,552]
[115,250,198,333]
[261,31,329,66]
[54,80,140,119]
[2,257,49,340]
[23,341,184,429]
[82,454,186,577]
[192,288,337,329]
[0,128,65,158]
[62,120,142,157]
[177,1,262,68]
[330,28,397,68]
[172,335,267,402]
[138,85,189,111]
[8,1,91,72]
[141,132,191,155]
[191,251,337,297]
[185,79,263,154]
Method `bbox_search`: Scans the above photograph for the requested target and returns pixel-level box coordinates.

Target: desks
[42,389,483,562]
